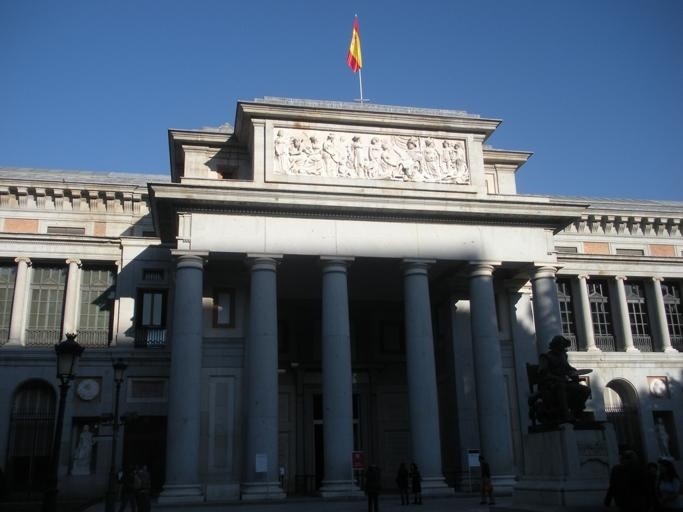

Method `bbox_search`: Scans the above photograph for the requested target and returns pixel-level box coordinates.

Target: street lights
[104,357,128,512]
[46,331,85,510]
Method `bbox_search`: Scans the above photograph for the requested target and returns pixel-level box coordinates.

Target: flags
[344,15,363,75]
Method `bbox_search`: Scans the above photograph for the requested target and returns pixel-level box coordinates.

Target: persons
[363,475,380,512]
[410,465,422,504]
[401,458,408,480]
[535,335,590,424]
[395,466,408,505]
[604,448,682,511]
[274,129,467,184]
[477,455,495,504]
[72,424,92,473]
[104,464,151,510]
[655,418,670,455]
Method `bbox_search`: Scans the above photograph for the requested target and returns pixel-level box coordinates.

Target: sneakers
[480,501,496,505]
[400,502,423,505]
